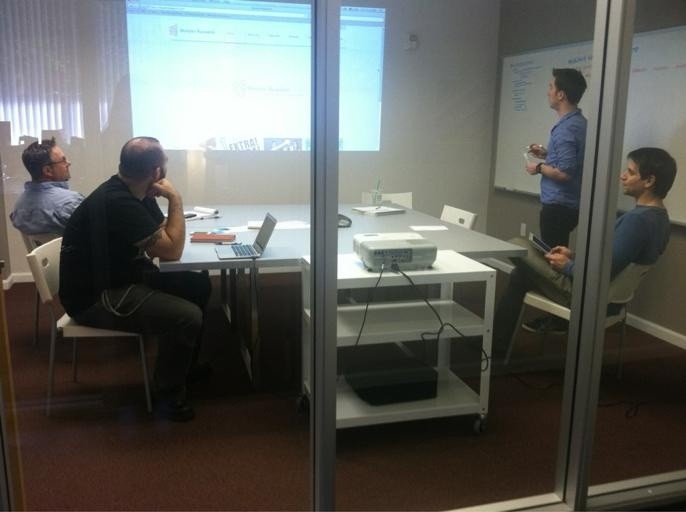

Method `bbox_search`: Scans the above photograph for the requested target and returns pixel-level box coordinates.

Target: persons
[8,136,84,235]
[521,67,588,334]
[58,137,213,419]
[482,147,678,365]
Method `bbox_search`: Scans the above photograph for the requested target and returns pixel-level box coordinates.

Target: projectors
[354,231,438,273]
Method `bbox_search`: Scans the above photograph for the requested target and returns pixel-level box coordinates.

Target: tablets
[529,231,550,254]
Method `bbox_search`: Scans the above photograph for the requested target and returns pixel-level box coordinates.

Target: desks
[160,202,528,394]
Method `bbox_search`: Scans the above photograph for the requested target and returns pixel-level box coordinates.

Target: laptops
[215,212,278,260]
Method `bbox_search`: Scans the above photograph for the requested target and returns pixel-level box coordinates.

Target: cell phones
[183,213,197,218]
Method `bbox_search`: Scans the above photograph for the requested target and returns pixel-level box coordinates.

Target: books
[190,232,236,243]
[184,206,219,223]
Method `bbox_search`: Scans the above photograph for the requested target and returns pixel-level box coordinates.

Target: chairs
[500,262,654,380]
[26,237,156,417]
[21,230,62,347]
[439,205,478,229]
[361,192,413,209]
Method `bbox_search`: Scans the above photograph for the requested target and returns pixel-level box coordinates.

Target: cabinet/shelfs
[298,249,498,435]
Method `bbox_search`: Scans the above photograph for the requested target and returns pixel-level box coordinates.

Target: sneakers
[522,316,568,336]
[150,383,195,421]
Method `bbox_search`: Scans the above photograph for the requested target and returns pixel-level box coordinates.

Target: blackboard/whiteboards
[494,25,686,229]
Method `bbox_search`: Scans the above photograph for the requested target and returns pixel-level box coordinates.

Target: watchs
[536,162,544,174]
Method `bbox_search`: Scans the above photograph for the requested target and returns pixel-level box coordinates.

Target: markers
[525,144,543,149]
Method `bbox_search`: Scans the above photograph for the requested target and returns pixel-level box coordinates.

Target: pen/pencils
[200,215,220,219]
[215,241,242,245]
